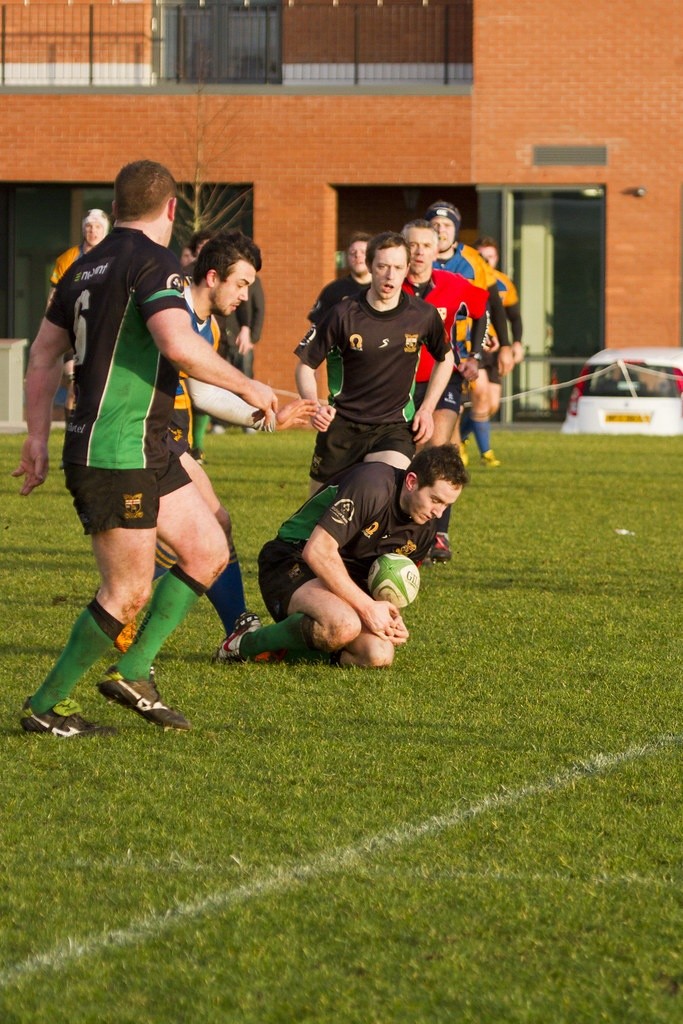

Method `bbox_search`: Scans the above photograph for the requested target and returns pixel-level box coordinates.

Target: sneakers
[429,532,452,564]
[250,646,290,665]
[190,448,206,464]
[209,613,263,664]
[458,444,469,467]
[20,696,121,740]
[480,449,501,468]
[96,666,190,732]
[115,618,138,652]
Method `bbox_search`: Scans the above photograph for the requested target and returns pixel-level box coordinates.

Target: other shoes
[242,426,257,433]
[208,424,226,435]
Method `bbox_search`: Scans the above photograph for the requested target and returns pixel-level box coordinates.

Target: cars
[559,347,682,438]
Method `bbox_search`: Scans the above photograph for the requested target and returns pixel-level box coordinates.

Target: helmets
[81,209,111,245]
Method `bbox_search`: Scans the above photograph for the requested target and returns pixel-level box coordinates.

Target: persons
[401,219,489,565]
[428,201,523,466]
[306,232,372,322]
[116,231,319,653]
[176,232,265,463]
[293,231,455,497]
[12,160,283,738]
[211,446,469,667]
[47,208,111,419]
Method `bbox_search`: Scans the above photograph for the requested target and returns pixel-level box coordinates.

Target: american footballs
[366,552,421,608]
